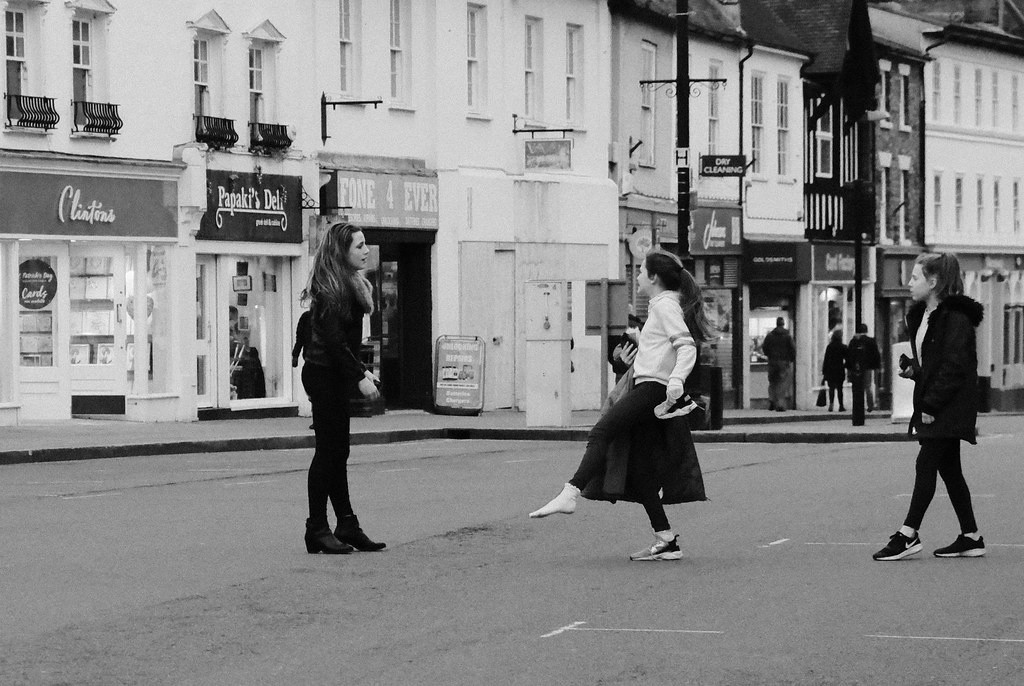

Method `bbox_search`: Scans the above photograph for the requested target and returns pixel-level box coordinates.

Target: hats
[858,324,868,332]
[777,317,785,325]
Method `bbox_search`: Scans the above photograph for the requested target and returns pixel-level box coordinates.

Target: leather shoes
[305,517,353,555]
[334,515,386,551]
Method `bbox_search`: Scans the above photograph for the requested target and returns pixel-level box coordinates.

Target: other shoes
[828,406,833,411]
[776,407,785,412]
[839,405,846,412]
[768,404,775,411]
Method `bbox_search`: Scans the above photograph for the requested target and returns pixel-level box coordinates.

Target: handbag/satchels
[815,378,827,406]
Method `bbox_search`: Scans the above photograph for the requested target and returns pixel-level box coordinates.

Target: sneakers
[872,530,923,561]
[933,532,986,558]
[654,392,697,419]
[630,533,684,561]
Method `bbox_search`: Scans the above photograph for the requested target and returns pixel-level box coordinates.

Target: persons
[872,252,987,562]
[300,222,388,556]
[823,330,848,412]
[529,248,716,560]
[761,316,796,412]
[292,311,314,428]
[848,324,882,412]
[230,306,266,399]
[827,300,842,341]
